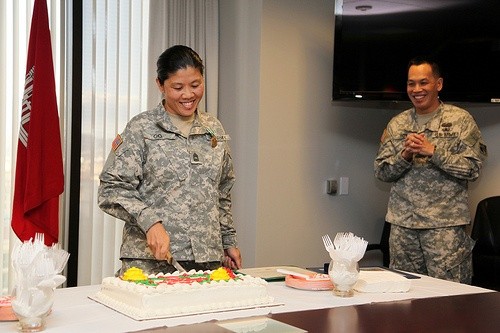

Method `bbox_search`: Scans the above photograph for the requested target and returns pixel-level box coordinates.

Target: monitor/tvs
[332,0,500,104]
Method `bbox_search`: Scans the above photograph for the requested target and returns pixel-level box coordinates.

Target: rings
[412,142,415,147]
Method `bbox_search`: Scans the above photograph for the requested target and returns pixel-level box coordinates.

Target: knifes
[166,252,186,273]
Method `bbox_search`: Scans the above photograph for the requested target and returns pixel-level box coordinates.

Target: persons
[98,46,242,276]
[373,60,485,285]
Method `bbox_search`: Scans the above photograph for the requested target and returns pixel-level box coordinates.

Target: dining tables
[0,265,500,333]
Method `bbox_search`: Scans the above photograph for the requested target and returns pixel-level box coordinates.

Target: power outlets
[339,177,349,195]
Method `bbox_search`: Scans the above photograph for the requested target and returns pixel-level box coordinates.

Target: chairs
[471,196,500,265]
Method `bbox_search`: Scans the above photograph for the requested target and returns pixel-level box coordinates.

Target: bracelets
[433,146,437,154]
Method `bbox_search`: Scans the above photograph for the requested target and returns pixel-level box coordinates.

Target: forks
[11,233,71,316]
[321,232,368,259]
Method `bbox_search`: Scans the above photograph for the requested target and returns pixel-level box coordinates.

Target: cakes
[95,267,272,318]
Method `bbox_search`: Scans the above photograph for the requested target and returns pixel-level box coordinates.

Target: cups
[329,260,360,297]
[12,286,55,333]
